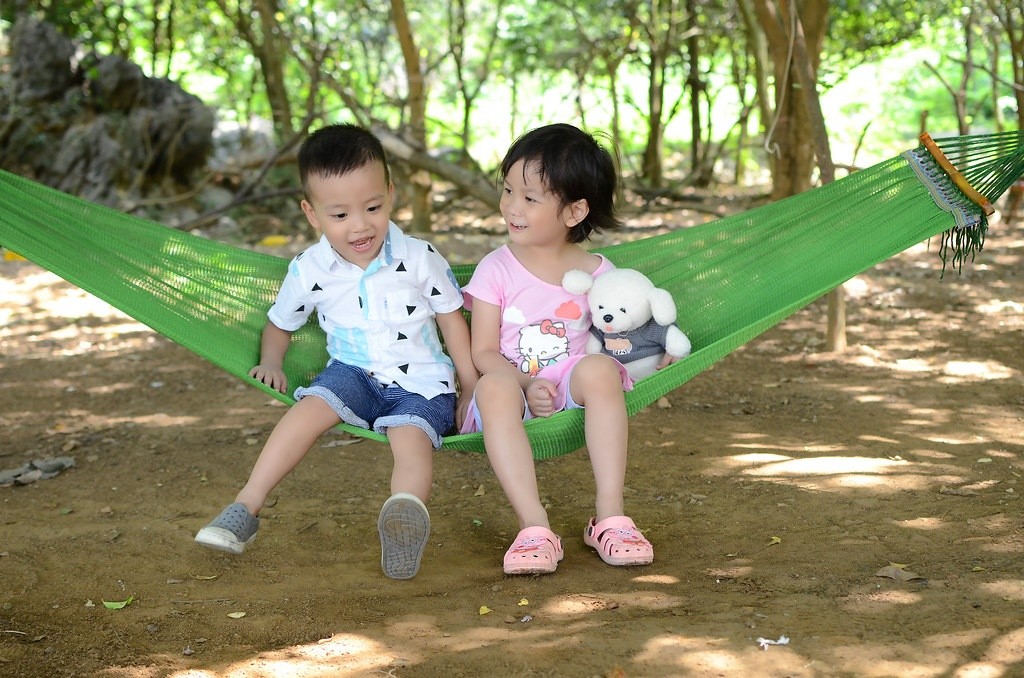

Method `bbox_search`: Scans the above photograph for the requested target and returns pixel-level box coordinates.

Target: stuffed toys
[562,270,692,382]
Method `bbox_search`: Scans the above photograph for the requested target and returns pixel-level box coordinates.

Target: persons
[194,125,479,580]
[462,122,652,575]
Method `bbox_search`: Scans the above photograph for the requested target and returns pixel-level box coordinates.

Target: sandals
[503,526,564,574]
[377,492,431,580]
[193,502,261,555]
[584,515,653,566]
